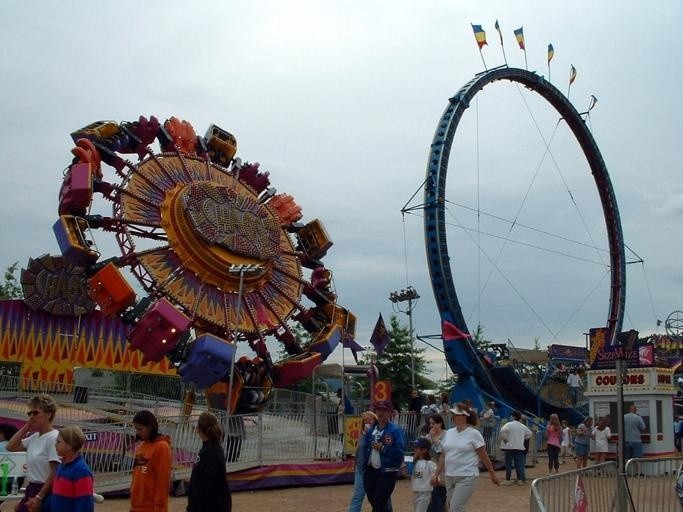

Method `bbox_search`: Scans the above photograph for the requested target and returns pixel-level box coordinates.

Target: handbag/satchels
[427,482,447,512]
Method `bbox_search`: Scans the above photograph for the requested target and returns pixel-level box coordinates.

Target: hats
[374,401,393,411]
[449,407,470,417]
[409,438,430,449]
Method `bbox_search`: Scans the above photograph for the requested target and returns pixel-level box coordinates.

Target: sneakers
[501,479,526,486]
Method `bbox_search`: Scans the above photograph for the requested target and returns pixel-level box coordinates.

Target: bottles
[2,460,10,495]
[11,476,18,495]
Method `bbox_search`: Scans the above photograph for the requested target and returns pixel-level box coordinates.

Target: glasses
[27,411,42,417]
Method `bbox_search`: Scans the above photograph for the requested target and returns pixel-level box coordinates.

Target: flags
[470,19,576,85]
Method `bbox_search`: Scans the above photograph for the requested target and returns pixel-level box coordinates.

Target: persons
[329,366,683,512]
[1,362,275,511]
